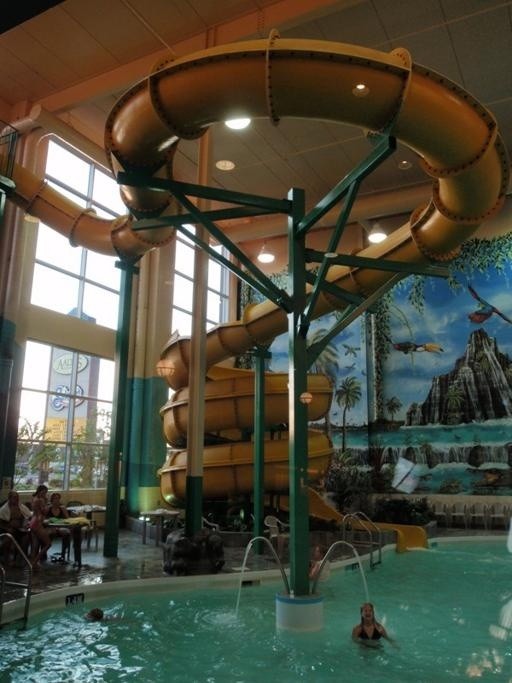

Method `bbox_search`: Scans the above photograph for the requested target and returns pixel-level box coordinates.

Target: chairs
[0,500,99,561]
[265,515,290,561]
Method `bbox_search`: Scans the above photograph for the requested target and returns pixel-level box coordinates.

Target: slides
[0,31,507,552]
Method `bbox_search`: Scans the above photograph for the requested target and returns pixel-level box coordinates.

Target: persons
[353,602,401,652]
[29,485,52,569]
[309,543,331,582]
[0,490,34,568]
[39,492,72,563]
[83,607,105,621]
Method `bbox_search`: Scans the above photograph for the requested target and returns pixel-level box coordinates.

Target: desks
[65,504,107,539]
[140,510,180,548]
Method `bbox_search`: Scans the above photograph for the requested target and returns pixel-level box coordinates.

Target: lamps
[367,220,388,244]
[257,239,276,264]
